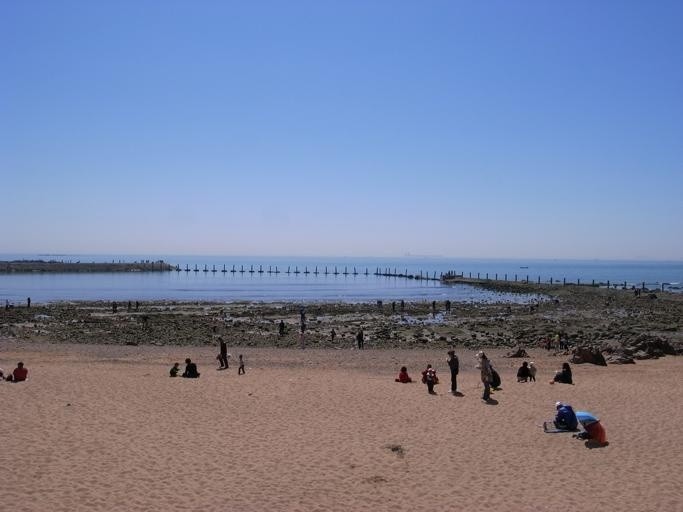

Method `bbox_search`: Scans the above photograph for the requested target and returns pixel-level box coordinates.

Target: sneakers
[481,397,489,403]
[448,389,456,393]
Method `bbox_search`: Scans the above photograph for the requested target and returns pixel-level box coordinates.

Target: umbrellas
[574,409,611,449]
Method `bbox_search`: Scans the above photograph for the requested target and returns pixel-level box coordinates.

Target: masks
[555,401,562,410]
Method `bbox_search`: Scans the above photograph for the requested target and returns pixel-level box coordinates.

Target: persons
[552,402,578,433]
[329,329,337,341]
[237,356,247,374]
[474,351,538,401]
[542,334,570,349]
[217,336,230,370]
[181,358,201,378]
[395,350,459,395]
[554,362,573,386]
[169,363,179,377]
[110,300,141,313]
[355,329,365,350]
[0,362,29,381]
[279,321,284,337]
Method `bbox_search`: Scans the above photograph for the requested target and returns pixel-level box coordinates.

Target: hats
[529,361,535,365]
[475,351,484,357]
